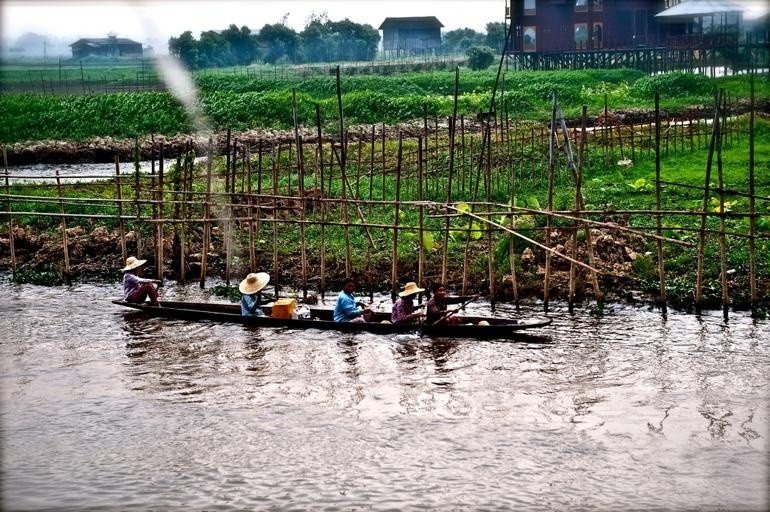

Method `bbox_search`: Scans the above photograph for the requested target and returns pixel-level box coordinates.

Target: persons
[238,271,271,316]
[392,282,427,324]
[120,256,159,306]
[333,276,371,323]
[427,283,480,325]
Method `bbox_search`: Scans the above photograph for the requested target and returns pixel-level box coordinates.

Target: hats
[398,282,426,297]
[123,256,147,271]
[238,272,270,295]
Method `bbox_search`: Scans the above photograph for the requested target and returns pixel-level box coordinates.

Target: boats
[112,299,553,336]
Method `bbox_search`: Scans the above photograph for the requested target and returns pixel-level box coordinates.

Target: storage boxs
[271,297,295,319]
